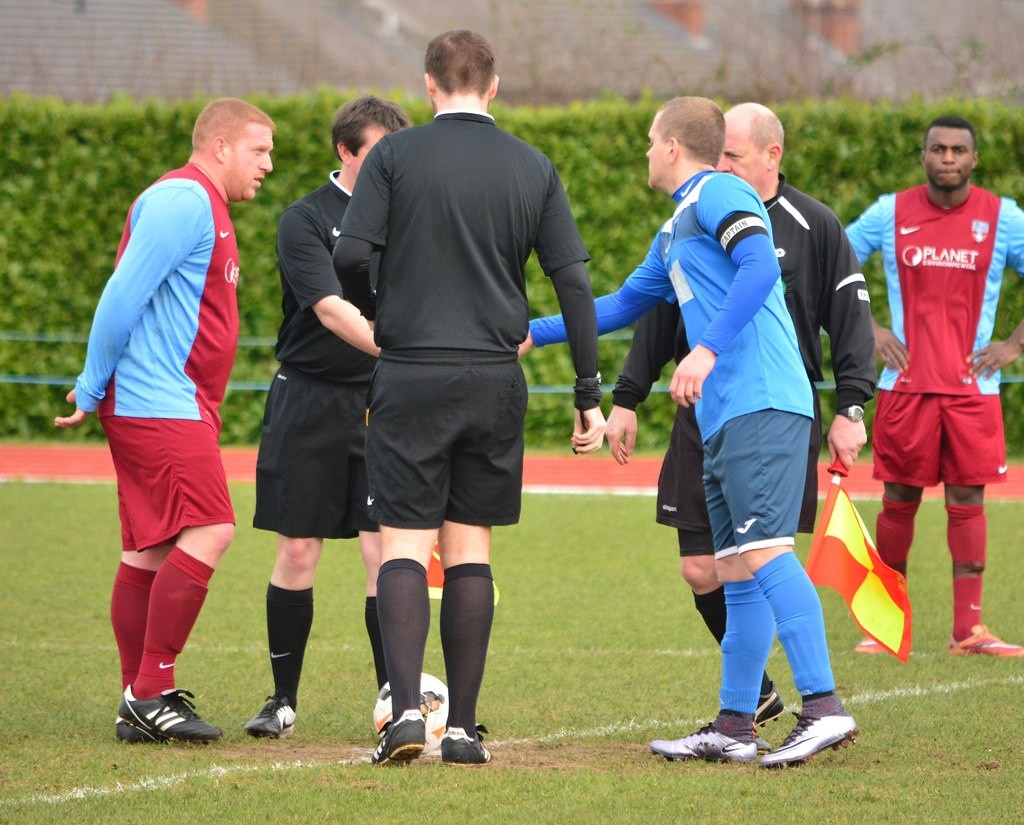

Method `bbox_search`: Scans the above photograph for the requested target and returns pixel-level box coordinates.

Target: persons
[332,26,607,766]
[53,98,274,742]
[606,102,876,732]
[514,96,861,770]
[842,115,1024,659]
[243,96,409,738]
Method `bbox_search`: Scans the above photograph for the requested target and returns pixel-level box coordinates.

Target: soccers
[372,672,449,755]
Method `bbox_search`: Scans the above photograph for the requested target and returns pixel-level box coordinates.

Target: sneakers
[761,712,859,768]
[115,717,209,745]
[854,637,886,653]
[756,737,771,754]
[373,710,425,767]
[649,722,757,764]
[244,694,296,740]
[947,623,1024,658]
[442,723,492,766]
[691,680,784,736]
[118,684,221,743]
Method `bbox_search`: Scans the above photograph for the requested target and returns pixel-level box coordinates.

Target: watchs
[839,405,864,423]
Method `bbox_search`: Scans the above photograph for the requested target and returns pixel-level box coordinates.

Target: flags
[805,482,913,663]
